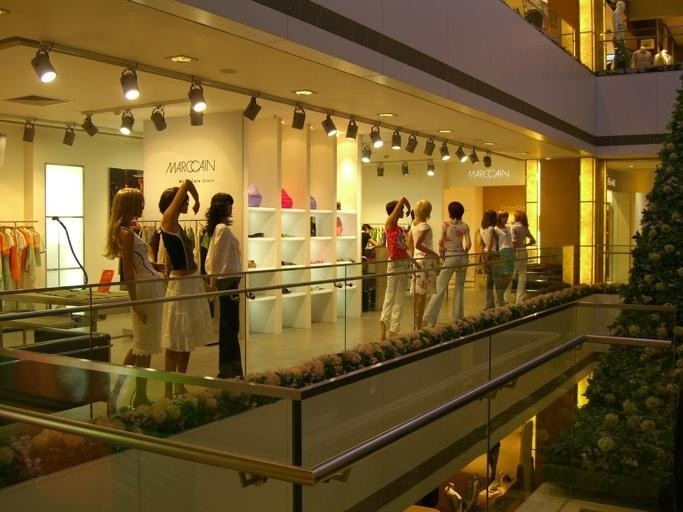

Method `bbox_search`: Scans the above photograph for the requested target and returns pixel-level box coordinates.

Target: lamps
[31,40,493,168]
[22,119,77,147]
[80,97,205,137]
[375,157,435,177]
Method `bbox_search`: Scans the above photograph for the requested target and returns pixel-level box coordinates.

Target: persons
[503,474,512,487]
[611,0,631,73]
[377,196,422,341]
[421,200,471,324]
[489,443,500,480]
[101,188,166,414]
[463,472,481,512]
[150,179,212,401]
[205,193,245,379]
[630,46,654,68]
[362,224,386,257]
[408,200,440,331]
[478,208,535,311]
[444,482,463,512]
[654,48,674,66]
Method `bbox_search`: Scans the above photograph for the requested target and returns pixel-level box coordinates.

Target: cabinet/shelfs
[240,111,363,336]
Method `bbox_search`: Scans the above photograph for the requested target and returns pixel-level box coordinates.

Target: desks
[1,289,133,340]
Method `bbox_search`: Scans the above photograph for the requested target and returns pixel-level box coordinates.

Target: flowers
[540,75,683,512]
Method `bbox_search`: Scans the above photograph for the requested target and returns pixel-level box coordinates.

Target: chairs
[0,326,114,427]
